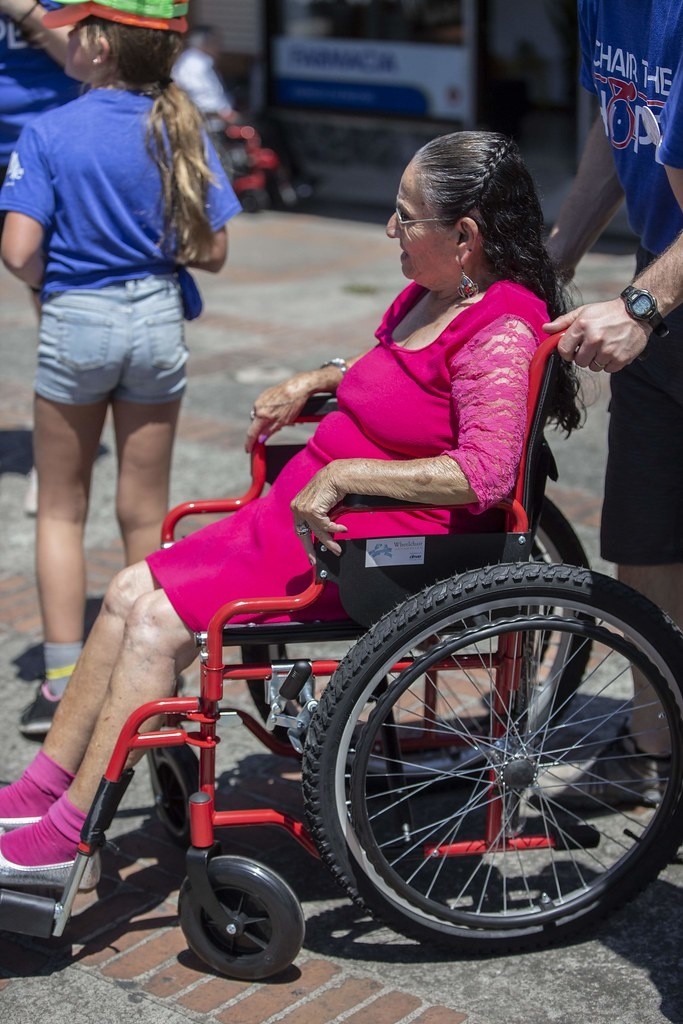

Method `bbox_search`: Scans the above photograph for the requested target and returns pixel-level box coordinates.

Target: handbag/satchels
[178,268,202,320]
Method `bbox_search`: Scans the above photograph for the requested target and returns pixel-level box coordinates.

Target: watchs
[620,286,670,338]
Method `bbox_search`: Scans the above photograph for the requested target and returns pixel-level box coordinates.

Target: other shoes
[534,718,674,809]
[17,680,61,736]
[0,787,103,889]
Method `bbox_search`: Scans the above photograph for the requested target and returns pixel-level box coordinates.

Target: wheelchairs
[199,111,292,213]
[1,326,682,984]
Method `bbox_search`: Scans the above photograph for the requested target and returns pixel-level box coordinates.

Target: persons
[0,0,246,733]
[526,0,683,814]
[0,131,602,890]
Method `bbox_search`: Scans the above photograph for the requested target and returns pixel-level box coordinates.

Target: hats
[41,0,190,35]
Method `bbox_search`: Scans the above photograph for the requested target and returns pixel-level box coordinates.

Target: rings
[593,360,605,368]
[249,410,255,420]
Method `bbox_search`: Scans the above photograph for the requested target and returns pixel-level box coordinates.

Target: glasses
[394,206,458,223]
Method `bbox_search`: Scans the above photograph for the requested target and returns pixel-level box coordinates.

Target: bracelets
[321,358,347,375]
[16,1,39,24]
